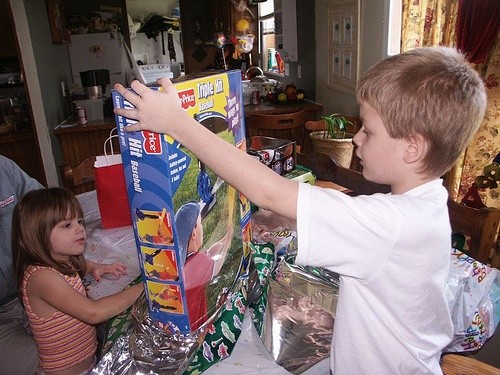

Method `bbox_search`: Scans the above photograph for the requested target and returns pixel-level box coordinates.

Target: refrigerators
[68,31,127,97]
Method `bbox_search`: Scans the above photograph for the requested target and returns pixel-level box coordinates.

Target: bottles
[266,49,272,71]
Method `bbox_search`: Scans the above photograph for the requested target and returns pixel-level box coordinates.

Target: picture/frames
[327,0,360,95]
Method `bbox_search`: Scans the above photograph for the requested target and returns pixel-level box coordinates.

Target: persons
[113,45,487,375]
[173,201,234,331]
[12,186,145,375]
[0,156,47,375]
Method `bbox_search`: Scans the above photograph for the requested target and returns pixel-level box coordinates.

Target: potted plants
[309,113,356,168]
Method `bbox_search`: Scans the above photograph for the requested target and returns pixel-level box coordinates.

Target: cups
[275,52,284,73]
[251,90,259,104]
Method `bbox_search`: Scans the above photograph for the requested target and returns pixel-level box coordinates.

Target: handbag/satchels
[93,126,134,230]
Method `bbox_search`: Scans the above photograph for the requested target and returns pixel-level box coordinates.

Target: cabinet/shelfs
[274,0,298,62]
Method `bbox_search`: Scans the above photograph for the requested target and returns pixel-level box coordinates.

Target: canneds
[75,107,88,125]
[252,91,261,104]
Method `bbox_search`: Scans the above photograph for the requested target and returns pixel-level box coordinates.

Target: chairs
[249,107,309,144]
[62,151,121,195]
[296,151,500,265]
[306,115,364,173]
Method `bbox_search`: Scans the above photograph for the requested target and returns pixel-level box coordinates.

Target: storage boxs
[247,136,316,213]
[111,68,252,336]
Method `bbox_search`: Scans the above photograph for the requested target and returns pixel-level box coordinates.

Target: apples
[266,85,304,101]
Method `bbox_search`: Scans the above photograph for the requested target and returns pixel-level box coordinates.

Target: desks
[54,99,322,195]
[76,189,141,301]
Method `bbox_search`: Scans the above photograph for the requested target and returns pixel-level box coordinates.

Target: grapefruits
[235,19,249,32]
